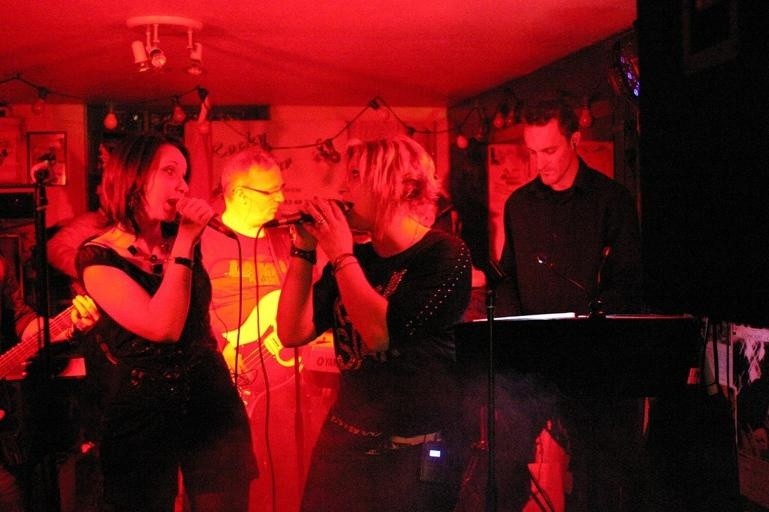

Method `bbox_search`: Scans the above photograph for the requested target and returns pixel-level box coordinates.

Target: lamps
[125,16,205,76]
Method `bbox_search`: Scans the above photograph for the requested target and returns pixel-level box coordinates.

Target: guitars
[228,325,304,415]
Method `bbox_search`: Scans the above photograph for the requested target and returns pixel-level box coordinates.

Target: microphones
[174,196,234,242]
[34,162,50,208]
[472,244,504,282]
[265,199,347,229]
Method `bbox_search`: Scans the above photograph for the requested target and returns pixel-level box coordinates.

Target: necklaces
[125,237,173,275]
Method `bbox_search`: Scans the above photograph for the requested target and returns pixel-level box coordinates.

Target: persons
[278,135,474,511]
[0,253,104,511]
[485,96,659,510]
[192,148,299,511]
[76,131,260,511]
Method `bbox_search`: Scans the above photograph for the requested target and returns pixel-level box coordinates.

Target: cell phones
[421,440,445,484]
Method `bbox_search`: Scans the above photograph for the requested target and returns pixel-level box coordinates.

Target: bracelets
[288,244,317,265]
[331,251,360,272]
[168,256,195,270]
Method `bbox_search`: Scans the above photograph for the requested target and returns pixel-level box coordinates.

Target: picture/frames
[25,129,70,188]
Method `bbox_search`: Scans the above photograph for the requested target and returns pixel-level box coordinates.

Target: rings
[318,218,326,224]
[81,314,90,319]
